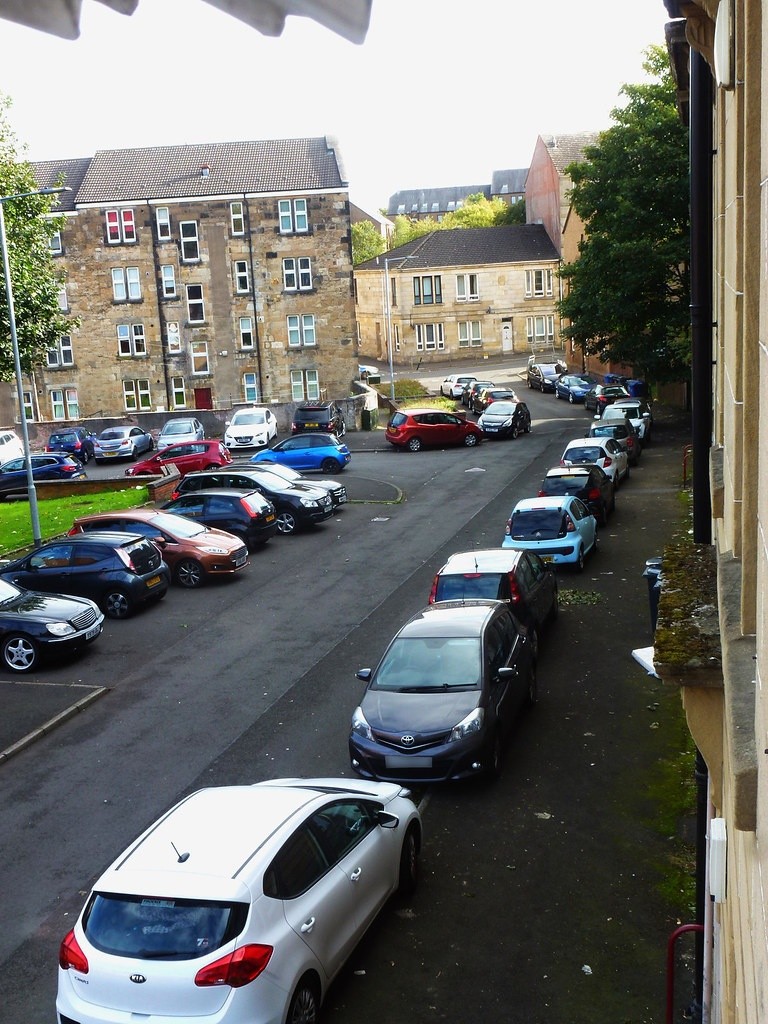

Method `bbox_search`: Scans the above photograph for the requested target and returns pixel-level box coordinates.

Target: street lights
[383,256,419,400]
[0,185,72,550]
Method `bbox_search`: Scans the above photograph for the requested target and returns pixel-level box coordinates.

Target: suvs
[290,400,347,438]
[170,467,335,536]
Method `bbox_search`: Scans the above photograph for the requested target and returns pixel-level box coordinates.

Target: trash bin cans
[642,558,662,631]
[603,373,627,390]
[627,380,647,397]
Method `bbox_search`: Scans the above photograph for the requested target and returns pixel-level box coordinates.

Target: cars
[500,496,599,573]
[0,430,25,466]
[43,426,98,465]
[0,576,107,675]
[526,361,569,393]
[359,364,379,380]
[537,463,616,529]
[53,774,426,1024]
[93,425,154,464]
[427,546,561,626]
[57,508,251,589]
[384,408,484,453]
[461,380,496,410]
[554,373,599,404]
[345,599,541,786]
[209,459,349,511]
[592,395,660,448]
[477,399,532,439]
[124,439,234,479]
[559,436,631,493]
[160,487,279,553]
[248,432,353,475]
[0,450,89,502]
[0,531,173,620]
[583,382,631,415]
[583,417,642,467]
[156,417,205,453]
[224,406,279,450]
[439,374,478,400]
[471,387,520,416]
